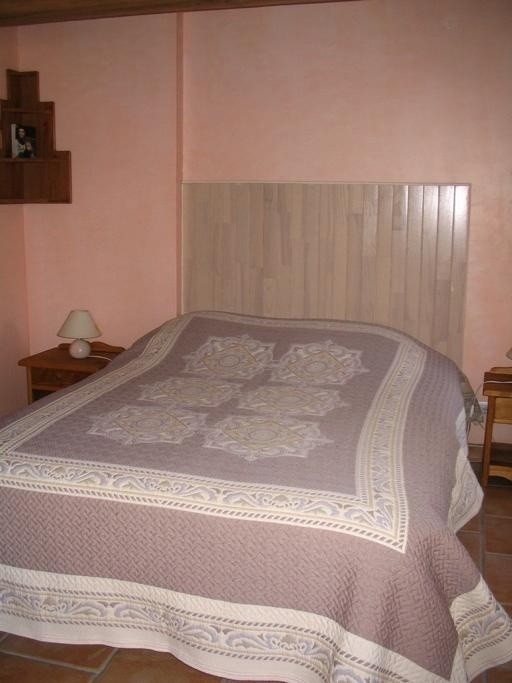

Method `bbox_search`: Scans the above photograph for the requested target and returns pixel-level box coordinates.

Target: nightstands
[483,362,511,482]
[18,340,127,405]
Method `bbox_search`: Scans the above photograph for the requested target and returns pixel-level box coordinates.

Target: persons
[16,128,33,156]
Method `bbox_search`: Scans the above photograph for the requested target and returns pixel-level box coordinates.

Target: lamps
[59,306,101,359]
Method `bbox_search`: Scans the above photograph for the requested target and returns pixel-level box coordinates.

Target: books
[11,124,37,158]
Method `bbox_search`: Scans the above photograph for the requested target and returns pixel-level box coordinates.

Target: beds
[1,310,473,679]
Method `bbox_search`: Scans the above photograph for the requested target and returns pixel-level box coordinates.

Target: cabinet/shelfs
[0,98,73,205]
